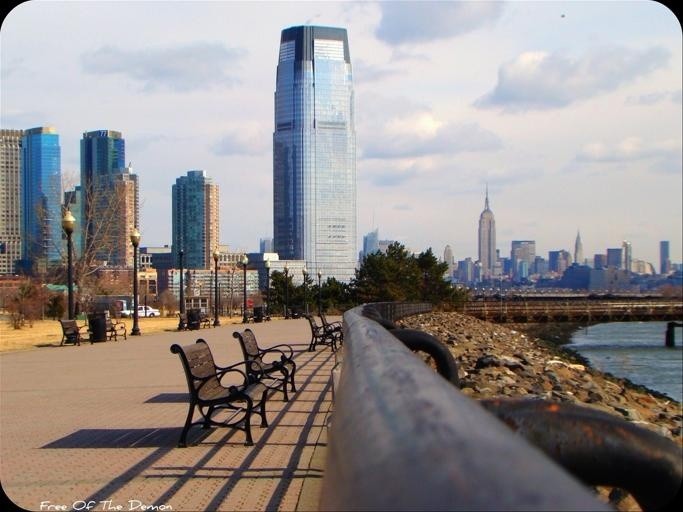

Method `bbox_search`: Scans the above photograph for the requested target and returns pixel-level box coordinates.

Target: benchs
[170,336,268,447]
[238,306,306,324]
[301,311,342,354]
[53,308,128,344]
[177,308,216,330]
[230,329,299,406]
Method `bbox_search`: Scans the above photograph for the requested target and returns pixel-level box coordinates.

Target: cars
[219,310,254,316]
[119,305,160,318]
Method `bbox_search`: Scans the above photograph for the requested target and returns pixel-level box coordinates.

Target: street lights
[301,265,307,316]
[129,224,141,336]
[283,261,290,319]
[241,255,249,323]
[317,269,321,316]
[212,245,220,326]
[177,248,186,330]
[448,285,679,301]
[61,207,75,344]
[264,257,270,320]
